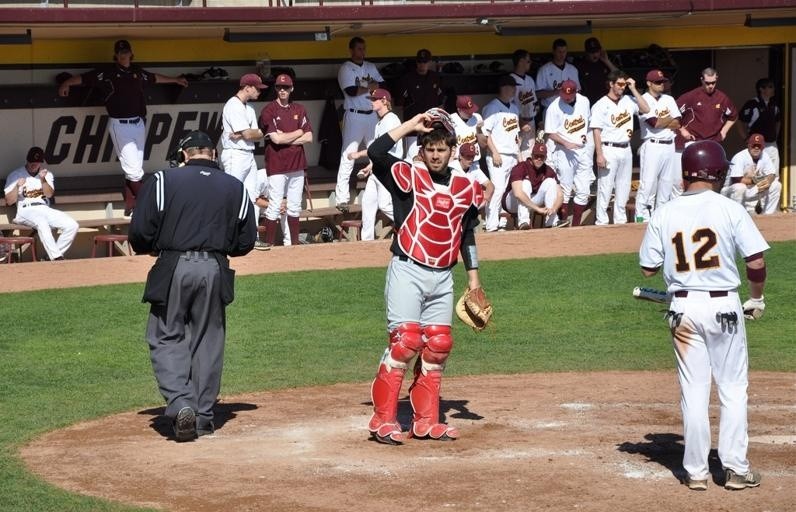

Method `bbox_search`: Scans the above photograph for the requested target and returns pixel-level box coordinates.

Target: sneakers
[370,431,401,445]
[724,469,761,490]
[253,240,270,250]
[335,202,348,214]
[172,407,195,441]
[196,421,214,435]
[551,220,569,229]
[518,222,528,230]
[682,471,708,490]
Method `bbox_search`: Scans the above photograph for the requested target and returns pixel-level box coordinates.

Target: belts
[119,118,139,123]
[22,202,45,207]
[398,254,448,271]
[674,290,727,297]
[604,142,628,148]
[179,252,216,259]
[349,108,372,114]
[650,138,672,144]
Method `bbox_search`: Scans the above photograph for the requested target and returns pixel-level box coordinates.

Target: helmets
[681,140,734,181]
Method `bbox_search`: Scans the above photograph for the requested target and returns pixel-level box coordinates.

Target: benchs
[262,205,364,221]
[0,215,128,234]
[302,177,369,196]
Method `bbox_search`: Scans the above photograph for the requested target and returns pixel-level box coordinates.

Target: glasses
[614,81,626,87]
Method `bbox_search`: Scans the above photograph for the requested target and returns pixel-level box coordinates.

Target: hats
[748,133,765,146]
[532,142,548,159]
[176,130,217,163]
[275,74,292,86]
[645,70,668,82]
[114,39,130,53]
[559,79,576,100]
[365,89,391,101]
[423,106,456,136]
[239,73,268,88]
[26,146,43,163]
[498,75,521,86]
[456,94,478,113]
[460,143,479,158]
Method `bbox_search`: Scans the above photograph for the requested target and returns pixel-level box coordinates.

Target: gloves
[742,295,765,321]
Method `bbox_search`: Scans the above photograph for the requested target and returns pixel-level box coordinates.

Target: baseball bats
[633,286,763,320]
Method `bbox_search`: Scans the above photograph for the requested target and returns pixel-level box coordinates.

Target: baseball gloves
[455,288,493,331]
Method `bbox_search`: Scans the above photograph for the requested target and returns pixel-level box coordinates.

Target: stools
[339,220,362,241]
[0,236,38,265]
[88,235,131,258]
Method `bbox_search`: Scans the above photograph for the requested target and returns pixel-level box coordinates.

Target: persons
[216,75,314,250]
[0,143,80,264]
[335,36,785,240]
[638,137,772,493]
[364,106,491,446]
[125,125,257,445]
[59,40,189,220]
